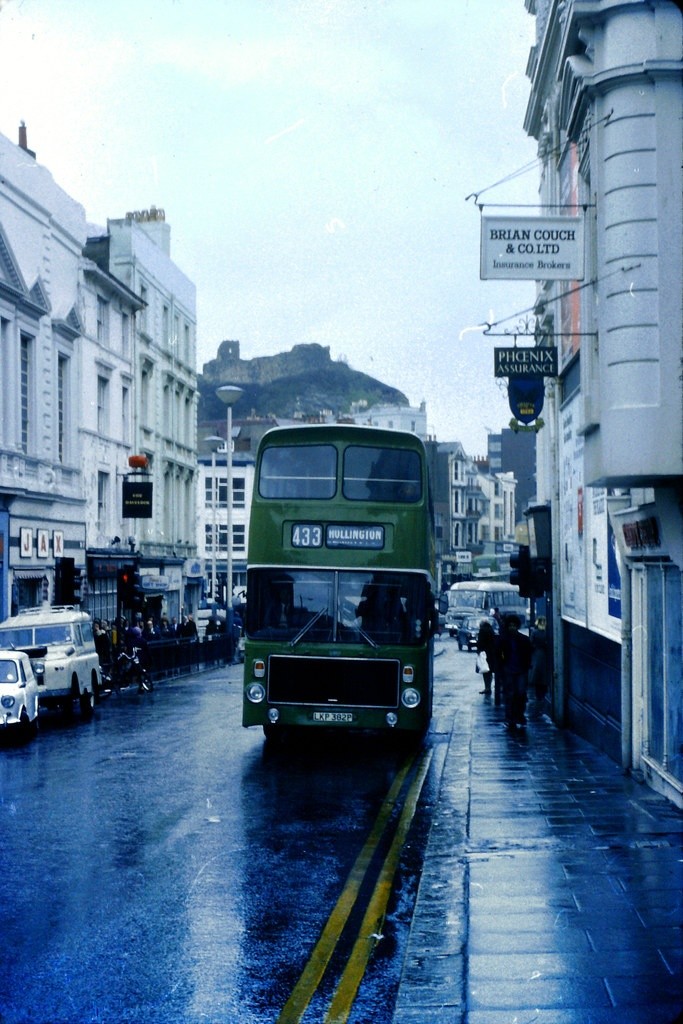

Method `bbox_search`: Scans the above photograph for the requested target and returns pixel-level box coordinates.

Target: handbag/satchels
[476,651,490,673]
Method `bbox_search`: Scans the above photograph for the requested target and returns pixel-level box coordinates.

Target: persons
[92,613,225,665]
[494,615,533,725]
[474,621,499,695]
[232,611,243,654]
[530,615,552,700]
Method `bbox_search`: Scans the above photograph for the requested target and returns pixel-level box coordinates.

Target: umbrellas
[207,615,224,621]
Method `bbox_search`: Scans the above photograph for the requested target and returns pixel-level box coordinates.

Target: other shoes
[478,689,492,695]
[516,716,526,724]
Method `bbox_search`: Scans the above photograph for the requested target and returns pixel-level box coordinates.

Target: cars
[0,650,41,741]
[436,608,502,653]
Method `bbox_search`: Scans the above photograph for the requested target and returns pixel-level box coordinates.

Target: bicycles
[100,647,154,700]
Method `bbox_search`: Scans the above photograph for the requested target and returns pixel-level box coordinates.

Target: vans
[0,604,104,719]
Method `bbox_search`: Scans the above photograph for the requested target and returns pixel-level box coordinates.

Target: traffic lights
[53,557,83,605]
[214,577,225,605]
[122,564,142,608]
[116,567,131,603]
[507,543,533,600]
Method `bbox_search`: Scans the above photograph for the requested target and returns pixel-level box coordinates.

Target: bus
[241,420,450,743]
[443,580,529,629]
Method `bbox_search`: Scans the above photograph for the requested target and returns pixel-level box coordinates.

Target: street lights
[214,385,246,663]
[203,434,225,633]
